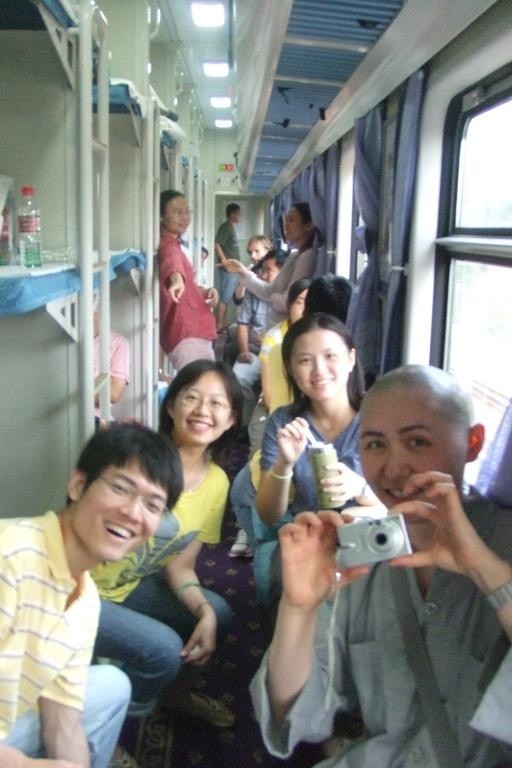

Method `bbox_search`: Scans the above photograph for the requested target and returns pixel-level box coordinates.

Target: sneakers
[172,687,235,728]
[229,529,253,559]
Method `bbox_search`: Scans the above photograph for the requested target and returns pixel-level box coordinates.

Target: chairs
[90,76,148,120]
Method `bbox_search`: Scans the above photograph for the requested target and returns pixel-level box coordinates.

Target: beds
[88,246,146,284]
[1,0,81,32]
[2,250,77,316]
[157,126,175,153]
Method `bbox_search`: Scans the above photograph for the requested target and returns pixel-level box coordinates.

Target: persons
[91,293,131,432]
[250,311,389,609]
[247,363,511,768]
[90,359,238,730]
[159,190,351,411]
[0,417,185,768]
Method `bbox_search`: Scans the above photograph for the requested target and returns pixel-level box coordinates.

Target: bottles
[2,192,13,265]
[308,442,348,511]
[14,186,47,268]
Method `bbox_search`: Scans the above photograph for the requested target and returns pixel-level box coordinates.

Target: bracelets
[269,464,294,480]
[484,577,512,612]
[174,581,202,601]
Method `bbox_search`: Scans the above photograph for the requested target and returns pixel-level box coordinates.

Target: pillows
[160,116,186,143]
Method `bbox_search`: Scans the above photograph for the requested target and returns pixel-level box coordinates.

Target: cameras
[337,513,413,569]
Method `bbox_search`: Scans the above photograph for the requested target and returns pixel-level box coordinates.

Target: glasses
[97,476,170,525]
